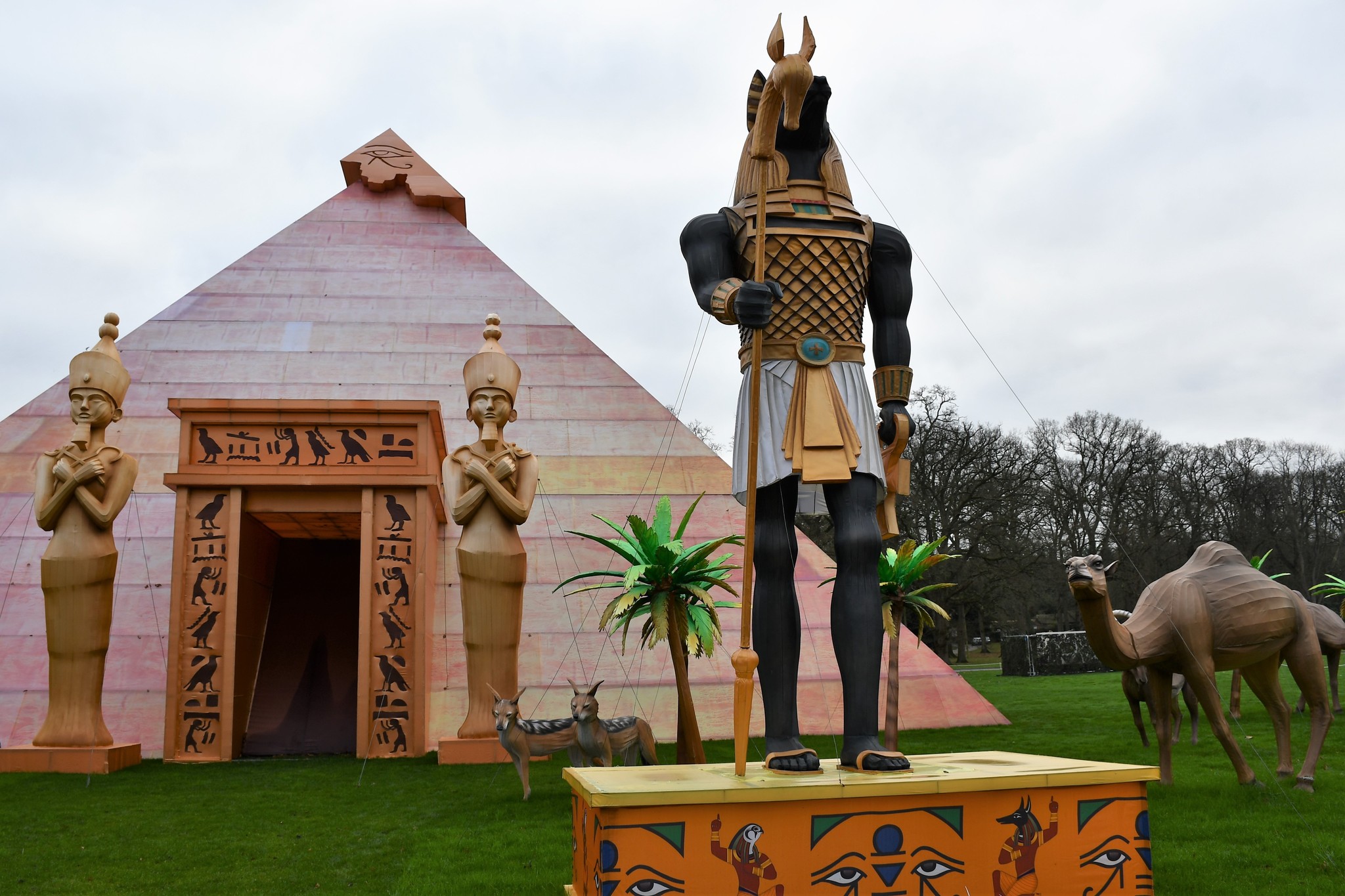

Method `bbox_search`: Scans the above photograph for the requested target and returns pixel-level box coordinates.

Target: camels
[1063,541,1345,797]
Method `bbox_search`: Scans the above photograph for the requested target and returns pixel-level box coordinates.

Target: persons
[32,313,138,747]
[441,313,540,740]
[679,71,917,771]
